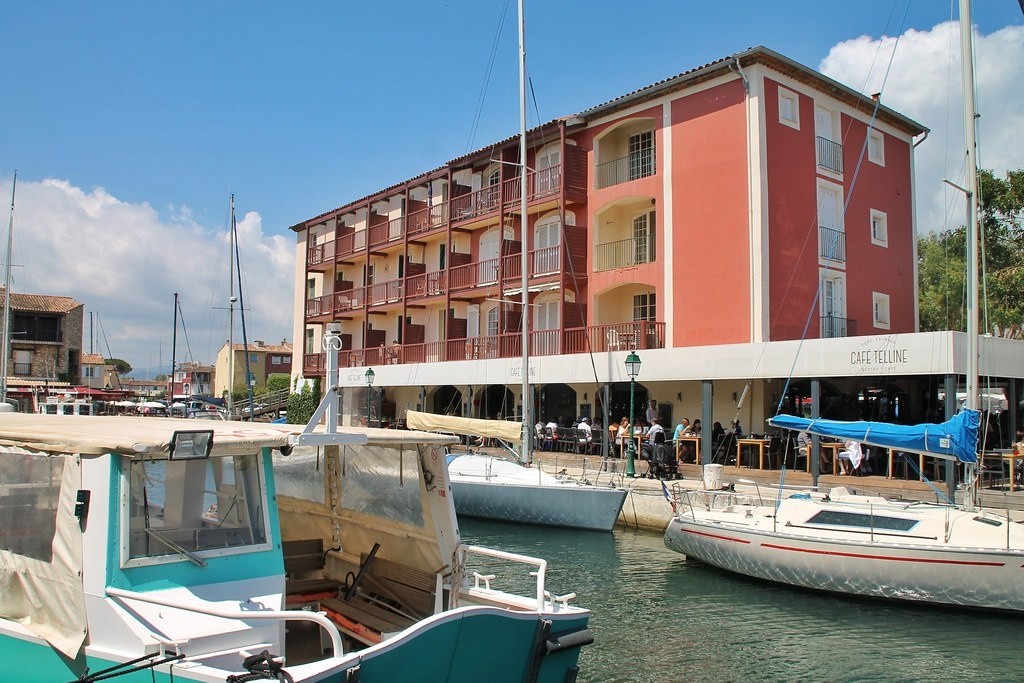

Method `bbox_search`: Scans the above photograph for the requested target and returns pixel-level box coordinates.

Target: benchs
[281,538,350,608]
[317,552,444,655]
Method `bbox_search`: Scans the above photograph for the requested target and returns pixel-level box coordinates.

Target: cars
[102,394,226,420]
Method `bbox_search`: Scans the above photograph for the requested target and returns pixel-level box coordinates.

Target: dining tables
[619,427,1024,494]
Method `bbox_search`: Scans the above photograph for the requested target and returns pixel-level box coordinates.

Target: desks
[466,343,485,359]
[419,214,441,229]
[619,331,635,350]
[364,417,392,427]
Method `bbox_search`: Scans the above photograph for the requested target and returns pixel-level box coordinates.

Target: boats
[655,2,1024,609]
[1,401,596,682]
[434,445,623,535]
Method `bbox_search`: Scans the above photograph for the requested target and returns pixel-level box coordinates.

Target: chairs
[457,206,472,222]
[338,295,350,312]
[532,426,627,459]
[607,329,621,352]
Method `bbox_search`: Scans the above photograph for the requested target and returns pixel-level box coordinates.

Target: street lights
[620,351,642,478]
[183,381,190,418]
[364,367,377,428]
[248,372,257,420]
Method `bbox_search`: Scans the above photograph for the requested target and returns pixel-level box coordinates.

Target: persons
[447,398,744,457]
[837,440,863,476]
[114,403,173,418]
[797,431,829,474]
[803,403,812,417]
[1011,434,1024,486]
